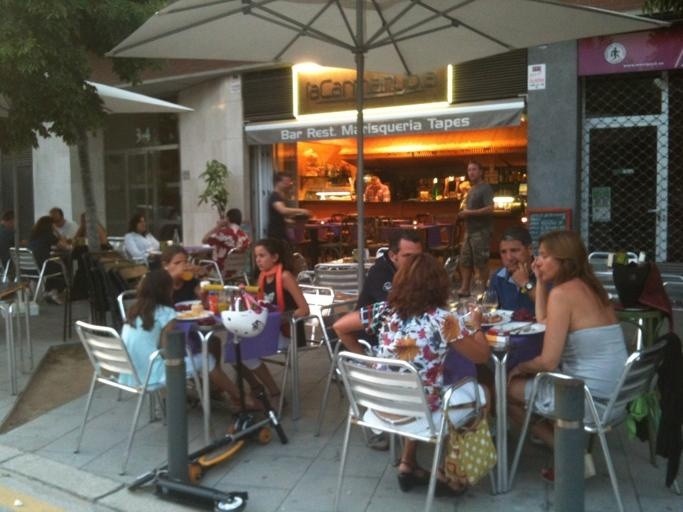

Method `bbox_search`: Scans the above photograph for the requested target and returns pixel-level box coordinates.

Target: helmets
[221,307,268,339]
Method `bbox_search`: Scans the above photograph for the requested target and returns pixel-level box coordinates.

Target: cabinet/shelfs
[481,164,529,212]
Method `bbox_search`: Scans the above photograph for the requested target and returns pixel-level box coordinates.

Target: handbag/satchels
[443,418,498,493]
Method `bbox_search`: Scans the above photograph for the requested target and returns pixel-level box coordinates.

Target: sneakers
[43,291,63,306]
[365,429,389,450]
[538,464,555,482]
[451,291,471,297]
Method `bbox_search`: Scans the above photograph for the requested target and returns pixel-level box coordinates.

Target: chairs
[72,284,334,475]
[0,216,682,396]
[318,297,683,512]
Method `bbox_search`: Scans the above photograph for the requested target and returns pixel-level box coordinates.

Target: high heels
[395,459,432,492]
[435,468,468,498]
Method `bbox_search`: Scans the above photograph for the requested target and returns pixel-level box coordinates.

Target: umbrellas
[105,0,674,302]
[0,73,196,281]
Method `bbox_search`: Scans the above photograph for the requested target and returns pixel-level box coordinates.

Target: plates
[174,299,201,309]
[175,310,214,321]
[488,320,546,336]
[481,308,513,326]
[284,212,313,221]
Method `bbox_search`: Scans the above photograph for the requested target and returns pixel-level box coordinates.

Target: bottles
[171,228,180,246]
[199,286,242,315]
[431,177,439,199]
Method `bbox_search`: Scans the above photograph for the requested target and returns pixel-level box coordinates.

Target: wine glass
[445,289,501,325]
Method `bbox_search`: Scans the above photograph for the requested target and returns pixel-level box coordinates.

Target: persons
[452,160,497,297]
[363,173,391,203]
[267,172,312,243]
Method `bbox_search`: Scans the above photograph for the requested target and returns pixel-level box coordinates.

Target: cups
[420,192,428,201]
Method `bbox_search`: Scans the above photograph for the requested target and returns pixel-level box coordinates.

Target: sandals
[229,384,290,412]
